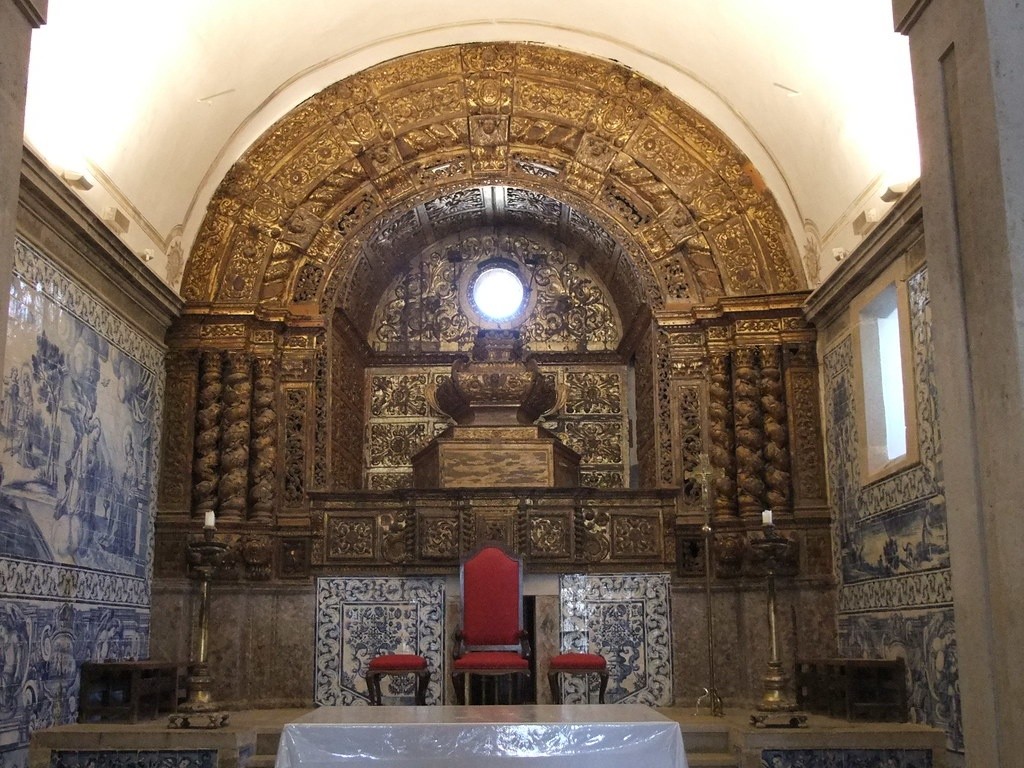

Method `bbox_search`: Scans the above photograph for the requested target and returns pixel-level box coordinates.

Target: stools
[792,657,910,725]
[548,652,609,705]
[76,659,179,726]
[366,654,431,707]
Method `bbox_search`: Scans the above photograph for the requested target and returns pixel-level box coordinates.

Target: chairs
[448,540,536,708]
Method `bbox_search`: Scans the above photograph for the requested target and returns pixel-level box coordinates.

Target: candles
[204,509,215,526]
[762,509,773,524]
